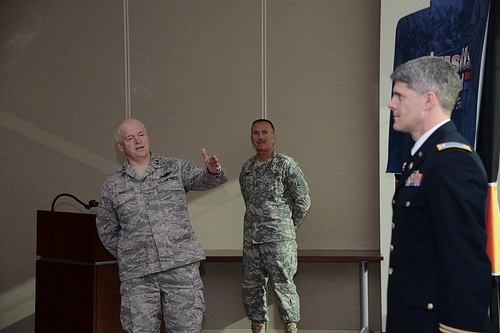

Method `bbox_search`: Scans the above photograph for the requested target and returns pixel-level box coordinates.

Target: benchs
[204,250,381,333]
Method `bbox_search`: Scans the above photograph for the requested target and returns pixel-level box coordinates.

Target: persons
[239,119,311,333]
[96,119,228,333]
[386,56,494,333]
